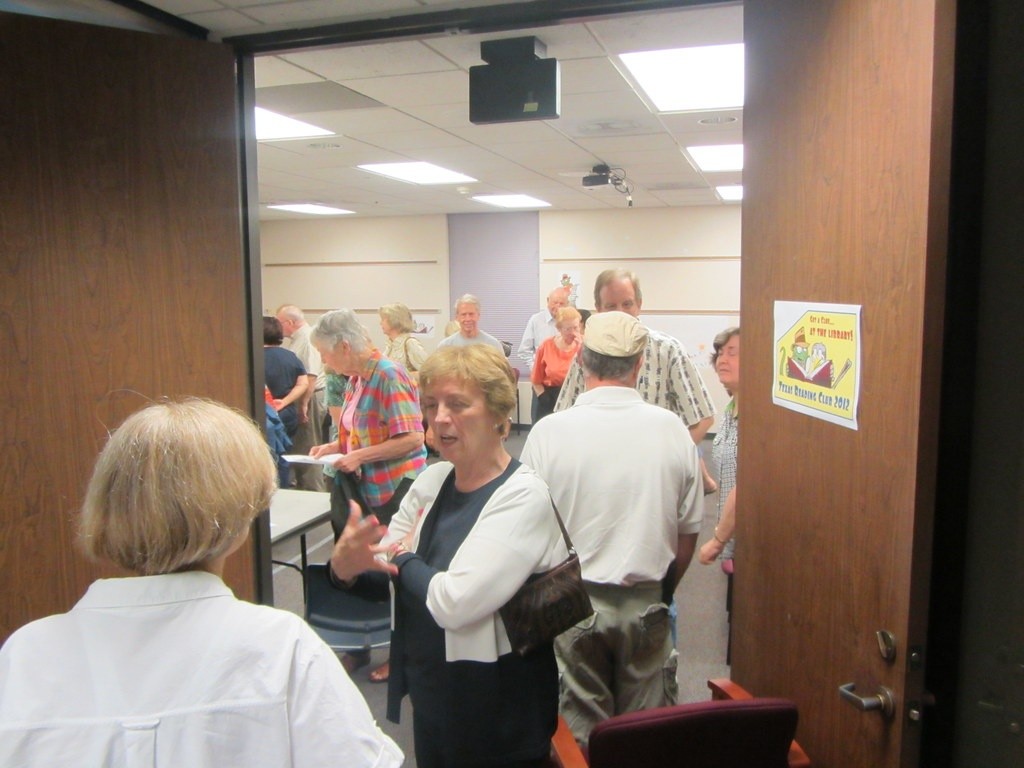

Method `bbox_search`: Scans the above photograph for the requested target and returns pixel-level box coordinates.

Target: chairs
[551,677,812,768]
[294,529,395,656]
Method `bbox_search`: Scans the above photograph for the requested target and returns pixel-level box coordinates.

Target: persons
[264,267,739,768]
[0,395,406,768]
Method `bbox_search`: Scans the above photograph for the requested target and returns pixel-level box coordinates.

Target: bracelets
[712,526,731,545]
[386,542,409,564]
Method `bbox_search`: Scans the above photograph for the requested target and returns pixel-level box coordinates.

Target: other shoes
[369,659,389,683]
[340,652,360,676]
[288,478,298,487]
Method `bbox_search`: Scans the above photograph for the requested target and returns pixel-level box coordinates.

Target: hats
[584,311,650,358]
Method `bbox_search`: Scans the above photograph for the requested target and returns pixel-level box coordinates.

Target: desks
[264,487,338,612]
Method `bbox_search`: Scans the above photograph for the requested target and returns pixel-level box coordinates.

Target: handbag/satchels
[498,491,595,656]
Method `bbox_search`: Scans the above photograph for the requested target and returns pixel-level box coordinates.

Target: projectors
[581,175,627,205]
